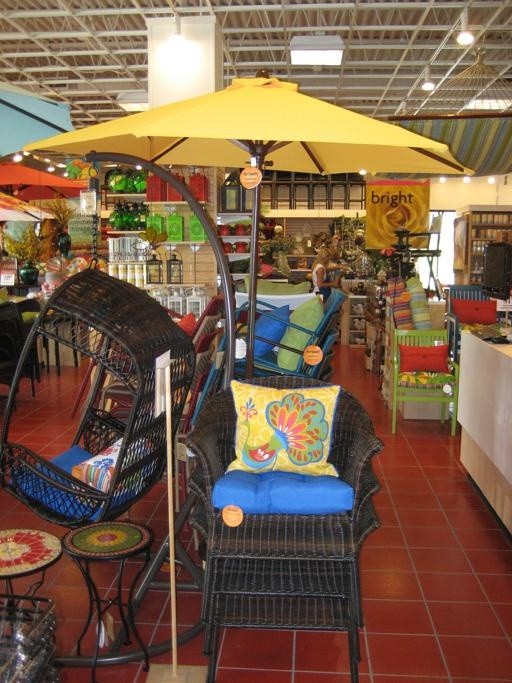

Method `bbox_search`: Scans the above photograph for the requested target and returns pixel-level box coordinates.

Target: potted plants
[45,195,76,258]
[4,224,42,285]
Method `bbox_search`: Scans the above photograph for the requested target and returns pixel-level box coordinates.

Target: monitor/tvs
[481,242,512,301]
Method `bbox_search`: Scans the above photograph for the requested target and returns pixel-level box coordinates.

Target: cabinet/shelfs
[453,204,512,285]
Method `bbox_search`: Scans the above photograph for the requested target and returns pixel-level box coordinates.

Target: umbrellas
[20,68,478,383]
[0,160,88,203]
[0,189,57,224]
[1,86,81,158]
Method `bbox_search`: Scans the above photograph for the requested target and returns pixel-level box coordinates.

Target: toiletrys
[108,167,208,324]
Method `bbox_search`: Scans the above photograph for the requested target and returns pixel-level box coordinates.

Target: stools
[0,286,80,396]
[0,520,155,683]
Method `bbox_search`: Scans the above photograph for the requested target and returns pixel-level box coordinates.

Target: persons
[305,246,354,281]
[311,251,343,300]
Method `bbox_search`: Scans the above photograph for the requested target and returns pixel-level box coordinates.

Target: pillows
[397,343,451,375]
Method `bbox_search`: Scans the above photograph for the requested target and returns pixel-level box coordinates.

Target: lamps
[420,7,473,92]
[286,34,346,67]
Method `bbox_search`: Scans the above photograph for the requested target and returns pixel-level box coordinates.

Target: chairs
[68,295,226,484]
[391,329,459,436]
